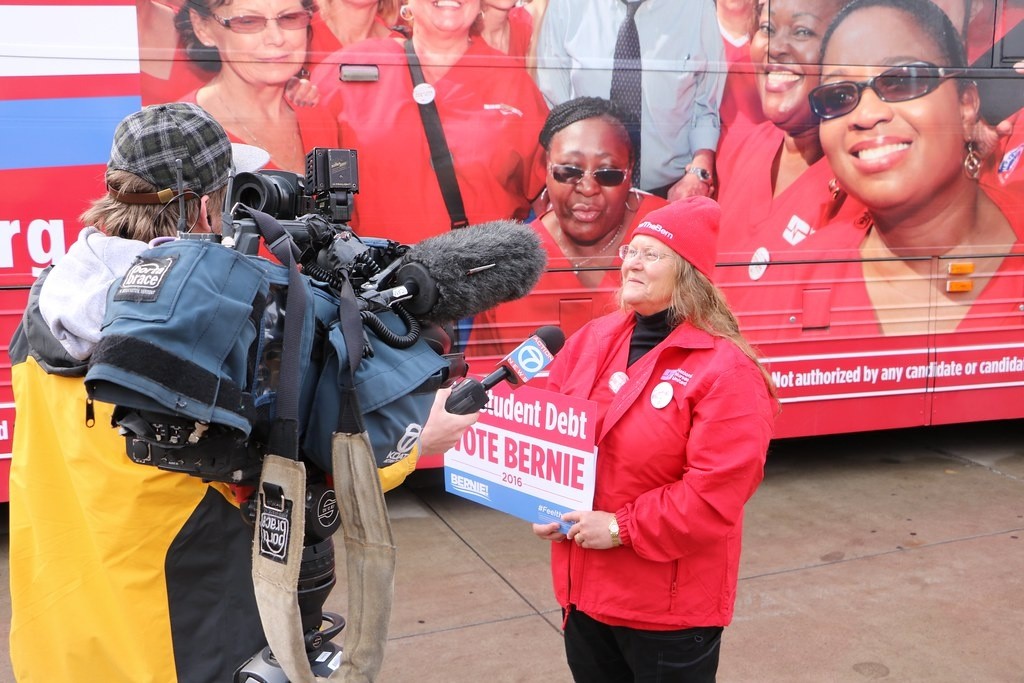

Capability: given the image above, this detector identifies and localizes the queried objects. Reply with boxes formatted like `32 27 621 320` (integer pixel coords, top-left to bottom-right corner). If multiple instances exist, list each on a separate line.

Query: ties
610 0 645 191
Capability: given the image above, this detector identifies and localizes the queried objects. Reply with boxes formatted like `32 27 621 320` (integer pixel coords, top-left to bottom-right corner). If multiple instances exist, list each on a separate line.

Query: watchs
609 514 620 547
688 167 713 188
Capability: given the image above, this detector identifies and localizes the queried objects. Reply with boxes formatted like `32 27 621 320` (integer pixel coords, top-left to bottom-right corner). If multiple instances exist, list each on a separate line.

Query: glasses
547 158 629 186
209 12 314 34
619 244 678 265
808 61 964 120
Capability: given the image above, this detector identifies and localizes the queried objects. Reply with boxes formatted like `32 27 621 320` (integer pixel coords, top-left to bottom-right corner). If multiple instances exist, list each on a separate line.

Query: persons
533 196 782 683
9 101 480 683
137 0 1024 360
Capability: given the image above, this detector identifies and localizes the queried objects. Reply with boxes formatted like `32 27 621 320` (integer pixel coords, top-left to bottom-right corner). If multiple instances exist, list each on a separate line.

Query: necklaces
213 76 297 170
558 225 621 273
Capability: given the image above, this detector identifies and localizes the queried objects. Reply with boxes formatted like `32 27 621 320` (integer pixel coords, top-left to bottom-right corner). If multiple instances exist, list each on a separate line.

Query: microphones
446 327 565 416
393 218 548 327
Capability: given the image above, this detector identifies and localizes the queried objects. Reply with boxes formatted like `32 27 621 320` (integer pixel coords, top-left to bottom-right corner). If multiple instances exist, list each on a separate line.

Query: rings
576 533 582 543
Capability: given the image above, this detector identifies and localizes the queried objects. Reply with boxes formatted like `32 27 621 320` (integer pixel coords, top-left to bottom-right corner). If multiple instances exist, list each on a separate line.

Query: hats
633 195 722 284
104 102 270 205
